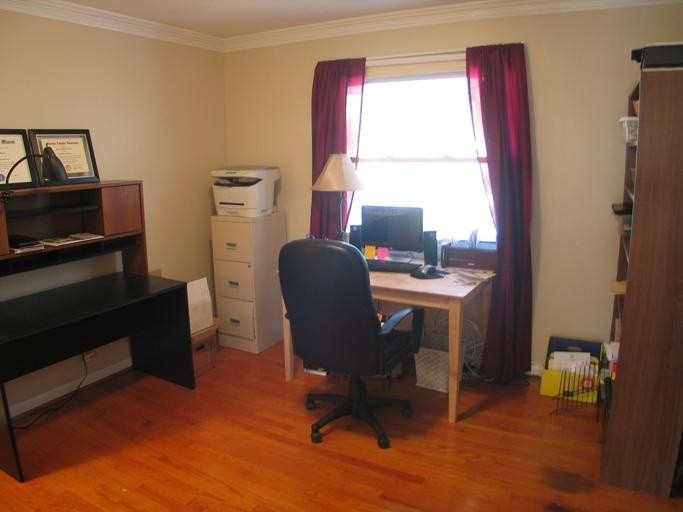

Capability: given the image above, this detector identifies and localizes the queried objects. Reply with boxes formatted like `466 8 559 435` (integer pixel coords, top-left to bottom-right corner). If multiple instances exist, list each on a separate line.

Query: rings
437 270 449 274
307 232 328 240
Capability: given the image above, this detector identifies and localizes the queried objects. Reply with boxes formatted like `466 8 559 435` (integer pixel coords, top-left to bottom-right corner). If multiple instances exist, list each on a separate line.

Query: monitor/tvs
9 231 104 254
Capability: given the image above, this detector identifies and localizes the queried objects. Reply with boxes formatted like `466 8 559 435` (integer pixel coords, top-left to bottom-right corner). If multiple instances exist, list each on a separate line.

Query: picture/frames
0 270 195 481
280 256 494 423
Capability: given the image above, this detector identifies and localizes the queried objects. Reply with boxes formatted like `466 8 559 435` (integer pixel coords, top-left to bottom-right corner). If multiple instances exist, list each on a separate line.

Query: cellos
211 166 280 218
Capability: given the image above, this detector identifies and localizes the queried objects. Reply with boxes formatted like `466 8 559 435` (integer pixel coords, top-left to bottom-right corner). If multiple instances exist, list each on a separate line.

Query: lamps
278 231 425 447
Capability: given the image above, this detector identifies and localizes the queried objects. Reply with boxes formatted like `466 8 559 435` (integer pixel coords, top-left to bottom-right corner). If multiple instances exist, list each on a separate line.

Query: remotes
421 265 436 275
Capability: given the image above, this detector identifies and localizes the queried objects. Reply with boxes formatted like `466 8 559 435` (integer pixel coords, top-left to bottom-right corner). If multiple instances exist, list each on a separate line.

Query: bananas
364 259 421 273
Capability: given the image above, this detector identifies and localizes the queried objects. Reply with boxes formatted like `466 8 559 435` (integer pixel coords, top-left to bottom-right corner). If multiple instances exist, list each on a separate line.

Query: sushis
8 234 38 249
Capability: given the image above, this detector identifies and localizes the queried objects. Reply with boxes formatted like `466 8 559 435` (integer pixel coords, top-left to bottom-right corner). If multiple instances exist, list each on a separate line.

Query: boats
423 231 437 267
349 225 361 252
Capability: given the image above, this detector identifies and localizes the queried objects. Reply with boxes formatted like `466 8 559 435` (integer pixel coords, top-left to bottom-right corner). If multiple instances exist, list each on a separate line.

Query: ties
361 206 423 262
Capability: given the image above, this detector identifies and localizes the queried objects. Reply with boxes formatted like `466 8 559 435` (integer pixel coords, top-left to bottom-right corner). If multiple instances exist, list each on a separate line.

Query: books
190 316 223 376
538 364 599 405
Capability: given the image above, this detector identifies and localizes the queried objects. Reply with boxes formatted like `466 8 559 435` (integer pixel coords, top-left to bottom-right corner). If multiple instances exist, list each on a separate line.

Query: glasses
313 153 357 238
2 148 65 191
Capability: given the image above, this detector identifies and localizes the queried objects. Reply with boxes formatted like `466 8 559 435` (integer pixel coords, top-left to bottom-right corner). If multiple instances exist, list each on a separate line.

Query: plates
209 214 286 356
600 67 683 499
0 179 149 279
